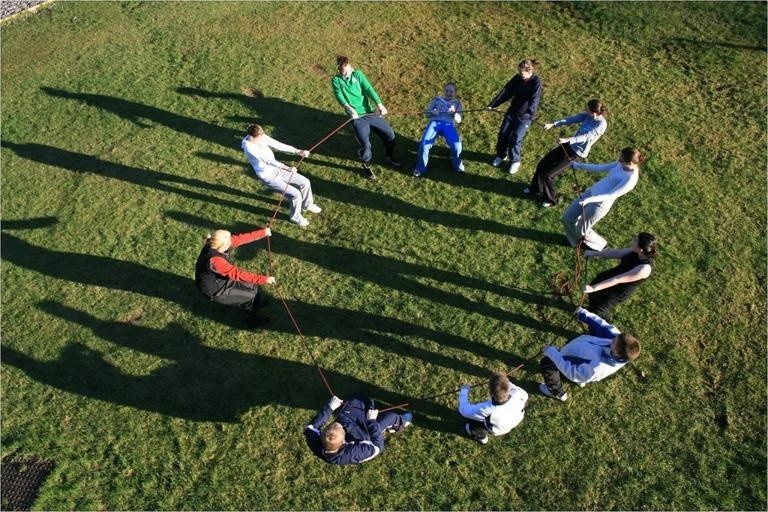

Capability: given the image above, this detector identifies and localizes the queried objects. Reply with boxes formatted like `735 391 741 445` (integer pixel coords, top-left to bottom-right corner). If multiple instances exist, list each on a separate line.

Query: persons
195 227 276 326
334 55 404 180
303 395 413 464
242 124 322 226
412 82 468 177
582 232 657 325
523 99 608 208
538 306 642 402
487 58 541 175
561 147 645 252
458 372 529 444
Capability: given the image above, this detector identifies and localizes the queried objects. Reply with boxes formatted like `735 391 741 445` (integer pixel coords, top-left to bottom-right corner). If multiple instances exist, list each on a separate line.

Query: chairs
384 157 401 167
287 216 309 229
362 164 378 181
538 383 567 401
491 155 508 168
302 205 323 214
464 423 489 444
508 162 523 175
402 414 413 431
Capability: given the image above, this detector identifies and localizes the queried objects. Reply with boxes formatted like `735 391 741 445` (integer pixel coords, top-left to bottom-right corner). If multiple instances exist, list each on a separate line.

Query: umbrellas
247 313 271 328
256 299 270 308
